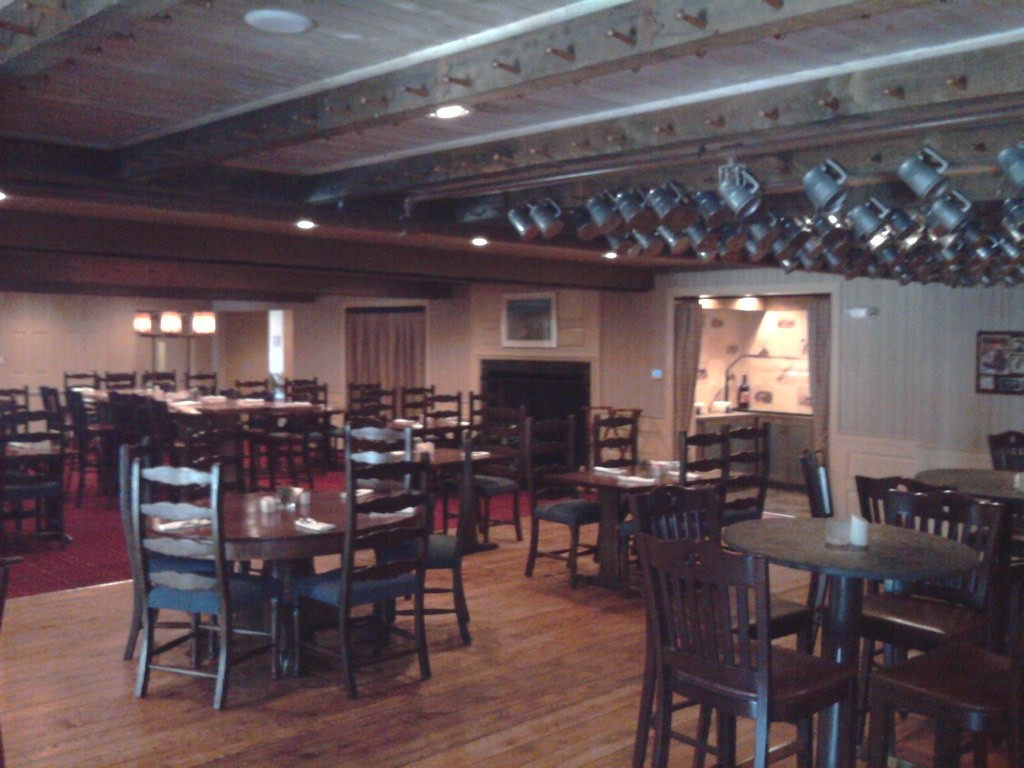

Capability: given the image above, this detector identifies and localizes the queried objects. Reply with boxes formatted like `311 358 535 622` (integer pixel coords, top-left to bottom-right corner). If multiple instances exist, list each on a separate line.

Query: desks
3 441 82 542
545 466 722 591
190 400 345 495
384 443 517 533
916 467 1024 653
723 518 978 768
143 484 425 678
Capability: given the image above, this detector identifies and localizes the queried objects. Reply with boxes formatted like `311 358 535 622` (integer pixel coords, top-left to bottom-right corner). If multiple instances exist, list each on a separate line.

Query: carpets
0 431 599 602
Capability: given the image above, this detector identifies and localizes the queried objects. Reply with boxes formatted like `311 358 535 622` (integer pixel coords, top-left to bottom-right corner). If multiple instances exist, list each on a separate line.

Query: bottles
737 374 750 409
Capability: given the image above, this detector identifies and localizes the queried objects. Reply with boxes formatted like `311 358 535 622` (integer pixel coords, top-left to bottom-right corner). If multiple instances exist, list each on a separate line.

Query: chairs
0 372 533 710
520 399 1024 767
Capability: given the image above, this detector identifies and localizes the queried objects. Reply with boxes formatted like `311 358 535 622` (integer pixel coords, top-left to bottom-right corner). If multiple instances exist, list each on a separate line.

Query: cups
825 516 850 549
850 515 869 546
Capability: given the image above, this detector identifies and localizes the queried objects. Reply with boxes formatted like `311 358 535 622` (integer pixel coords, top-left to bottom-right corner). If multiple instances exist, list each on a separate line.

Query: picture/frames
498 291 557 349
974 331 1024 396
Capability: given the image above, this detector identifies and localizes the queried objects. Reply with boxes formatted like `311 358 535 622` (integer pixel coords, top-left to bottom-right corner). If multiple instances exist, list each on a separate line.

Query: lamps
801 158 852 217
528 195 566 239
896 145 949 202
575 178 1024 288
506 207 537 240
849 197 889 239
718 165 762 223
132 309 215 338
932 189 972 234
996 146 1024 185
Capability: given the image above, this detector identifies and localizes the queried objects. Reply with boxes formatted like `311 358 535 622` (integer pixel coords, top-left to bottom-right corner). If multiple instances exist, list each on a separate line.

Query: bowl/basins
667 470 700 483
